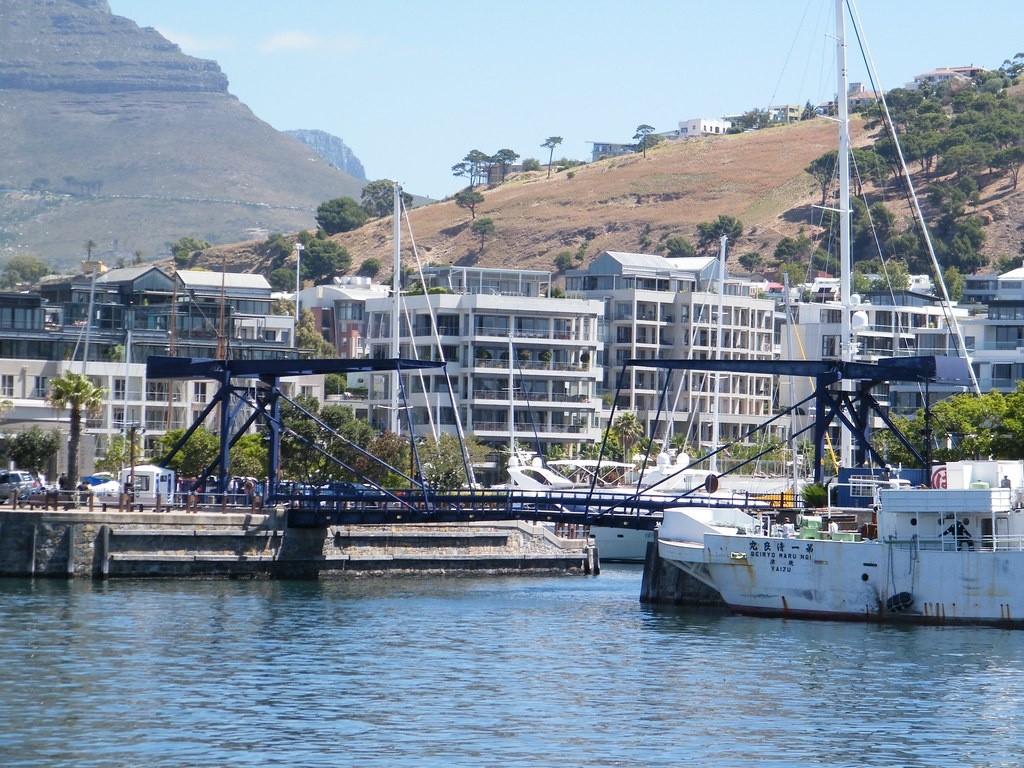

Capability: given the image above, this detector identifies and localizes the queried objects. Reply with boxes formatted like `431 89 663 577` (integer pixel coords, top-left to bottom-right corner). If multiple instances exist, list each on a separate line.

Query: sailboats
534 235 803 561
637 2 1023 623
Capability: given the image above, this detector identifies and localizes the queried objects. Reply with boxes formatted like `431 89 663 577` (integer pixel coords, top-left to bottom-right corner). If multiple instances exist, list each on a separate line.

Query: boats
0 461 387 509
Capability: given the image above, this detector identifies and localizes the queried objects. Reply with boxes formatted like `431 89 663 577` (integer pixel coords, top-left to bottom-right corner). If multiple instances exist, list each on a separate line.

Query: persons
777 518 791 538
58 471 68 489
938 520 976 551
877 467 891 491
827 517 838 541
762 518 771 536
77 480 93 505
